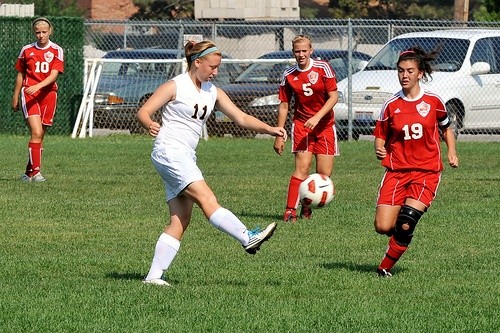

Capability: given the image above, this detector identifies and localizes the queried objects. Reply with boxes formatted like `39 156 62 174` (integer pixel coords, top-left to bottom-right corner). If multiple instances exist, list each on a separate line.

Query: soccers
298 173 337 209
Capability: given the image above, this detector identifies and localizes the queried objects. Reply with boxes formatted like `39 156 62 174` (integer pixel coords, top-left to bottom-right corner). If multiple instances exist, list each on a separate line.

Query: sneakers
21 172 46 182
284 211 296 222
142 279 170 287
242 222 277 255
300 207 311 220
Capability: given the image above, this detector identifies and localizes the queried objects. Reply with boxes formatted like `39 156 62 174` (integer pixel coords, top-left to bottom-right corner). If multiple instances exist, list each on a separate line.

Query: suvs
332 29 499 141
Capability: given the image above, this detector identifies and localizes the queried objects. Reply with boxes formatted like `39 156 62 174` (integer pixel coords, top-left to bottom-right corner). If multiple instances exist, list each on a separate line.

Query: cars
207 50 385 139
82 49 245 135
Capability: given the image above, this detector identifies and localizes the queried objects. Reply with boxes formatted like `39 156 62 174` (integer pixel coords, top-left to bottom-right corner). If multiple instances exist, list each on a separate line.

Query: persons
372 46 458 277
274 35 339 220
12 17 64 182
137 41 288 288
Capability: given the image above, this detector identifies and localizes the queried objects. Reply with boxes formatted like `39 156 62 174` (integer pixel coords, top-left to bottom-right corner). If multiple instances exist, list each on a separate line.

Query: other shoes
386 230 392 237
376 267 392 278
